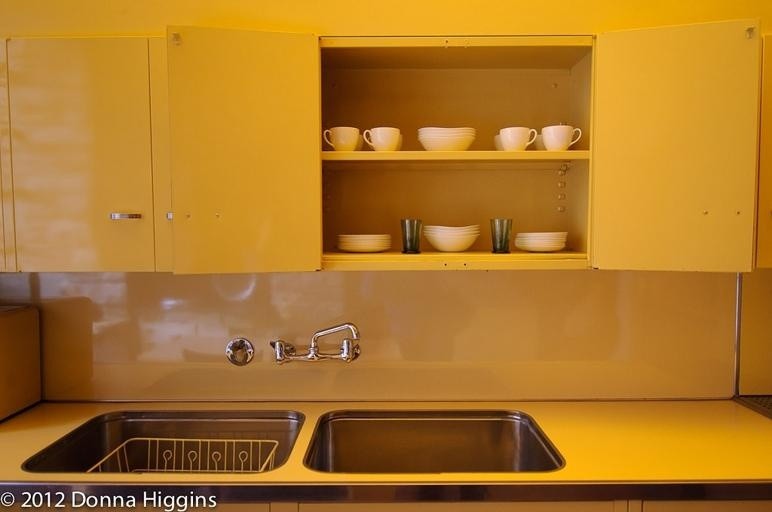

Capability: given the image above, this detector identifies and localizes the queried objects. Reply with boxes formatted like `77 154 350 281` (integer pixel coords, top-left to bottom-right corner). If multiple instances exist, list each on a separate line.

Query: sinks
299 407 568 475
20 409 307 477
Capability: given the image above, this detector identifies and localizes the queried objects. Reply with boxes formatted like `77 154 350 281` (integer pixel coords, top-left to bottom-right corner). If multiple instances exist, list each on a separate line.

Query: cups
490 216 513 255
534 134 546 151
541 124 583 153
322 125 360 151
366 134 403 151
354 135 363 152
400 217 423 254
362 127 400 152
494 134 504 150
499 126 537 151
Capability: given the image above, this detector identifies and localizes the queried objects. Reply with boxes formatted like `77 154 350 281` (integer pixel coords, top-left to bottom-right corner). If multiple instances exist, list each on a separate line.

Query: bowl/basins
423 221 481 253
416 125 476 151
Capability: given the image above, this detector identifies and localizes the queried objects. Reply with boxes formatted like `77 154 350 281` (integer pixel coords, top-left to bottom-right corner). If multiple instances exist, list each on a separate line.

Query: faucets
269 321 361 365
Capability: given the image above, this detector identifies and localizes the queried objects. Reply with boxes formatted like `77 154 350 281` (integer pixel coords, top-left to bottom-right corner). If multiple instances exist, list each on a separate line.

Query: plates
333 233 392 254
514 228 570 253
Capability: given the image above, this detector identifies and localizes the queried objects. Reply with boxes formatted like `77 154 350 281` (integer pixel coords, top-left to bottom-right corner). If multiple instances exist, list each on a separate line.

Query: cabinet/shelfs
153 19 763 273
5 39 172 273
756 30 770 270
